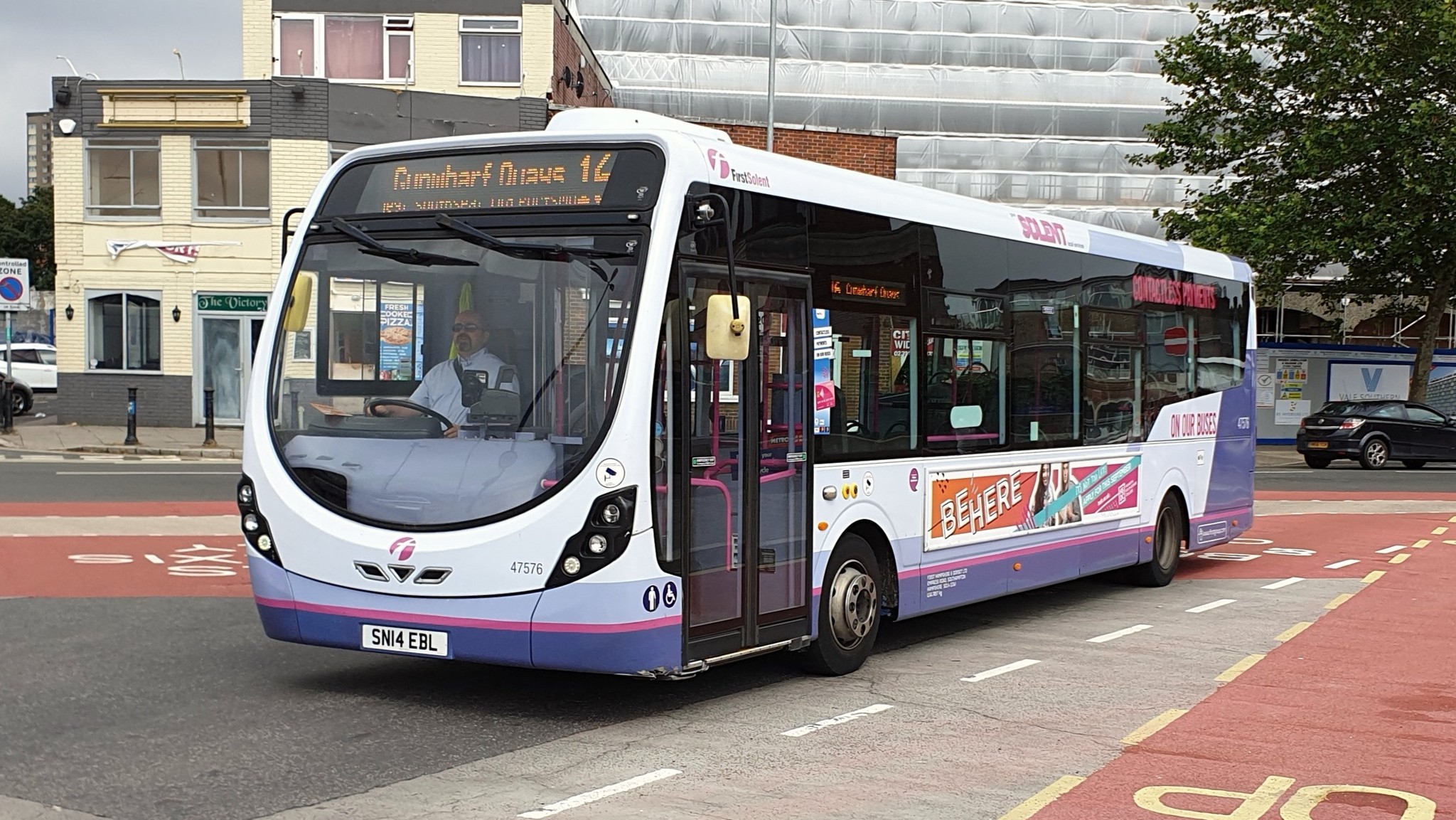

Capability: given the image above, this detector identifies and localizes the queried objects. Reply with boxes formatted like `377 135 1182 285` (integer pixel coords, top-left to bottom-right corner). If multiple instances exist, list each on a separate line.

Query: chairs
847 360 1211 440
484 328 531 427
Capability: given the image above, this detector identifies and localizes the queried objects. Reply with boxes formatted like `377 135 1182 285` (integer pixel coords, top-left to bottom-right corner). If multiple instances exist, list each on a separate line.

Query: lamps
558 67 582 88
272 80 306 93
580 54 588 69
66 304 74 320
173 305 181 322
56 77 73 107
572 71 597 98
59 118 77 134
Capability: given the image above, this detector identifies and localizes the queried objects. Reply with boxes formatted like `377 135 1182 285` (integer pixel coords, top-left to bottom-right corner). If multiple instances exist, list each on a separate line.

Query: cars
0 371 35 416
0 343 57 388
1296 400 1456 471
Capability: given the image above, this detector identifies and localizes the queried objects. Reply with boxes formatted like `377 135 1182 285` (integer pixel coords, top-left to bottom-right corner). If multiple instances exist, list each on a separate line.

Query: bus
233 108 1258 682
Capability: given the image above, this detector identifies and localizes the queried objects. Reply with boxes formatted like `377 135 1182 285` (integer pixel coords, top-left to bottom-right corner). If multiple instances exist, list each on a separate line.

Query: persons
366 307 521 437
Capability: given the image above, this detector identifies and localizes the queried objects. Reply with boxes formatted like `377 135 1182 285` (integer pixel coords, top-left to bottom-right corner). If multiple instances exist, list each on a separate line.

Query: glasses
452 323 478 333
